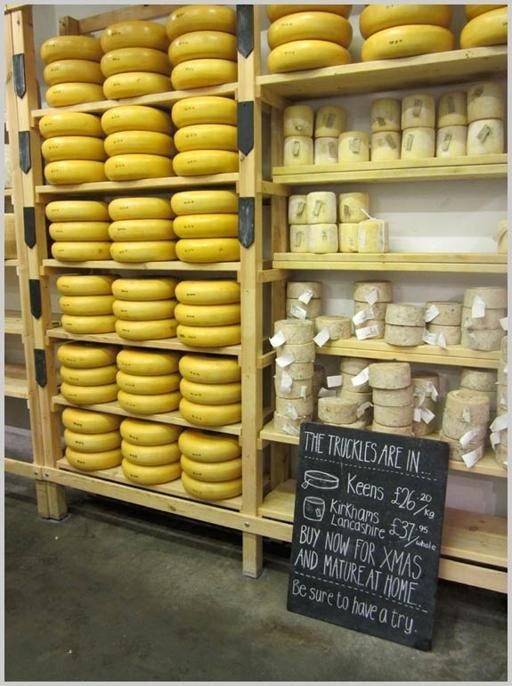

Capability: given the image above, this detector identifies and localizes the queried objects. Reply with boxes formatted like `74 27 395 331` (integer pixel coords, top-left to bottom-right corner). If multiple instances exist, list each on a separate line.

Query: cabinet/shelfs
1 1 245 578
245 1 509 602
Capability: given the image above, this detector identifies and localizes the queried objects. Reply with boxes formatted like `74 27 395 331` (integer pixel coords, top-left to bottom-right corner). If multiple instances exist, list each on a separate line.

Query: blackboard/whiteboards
286 422 450 652
